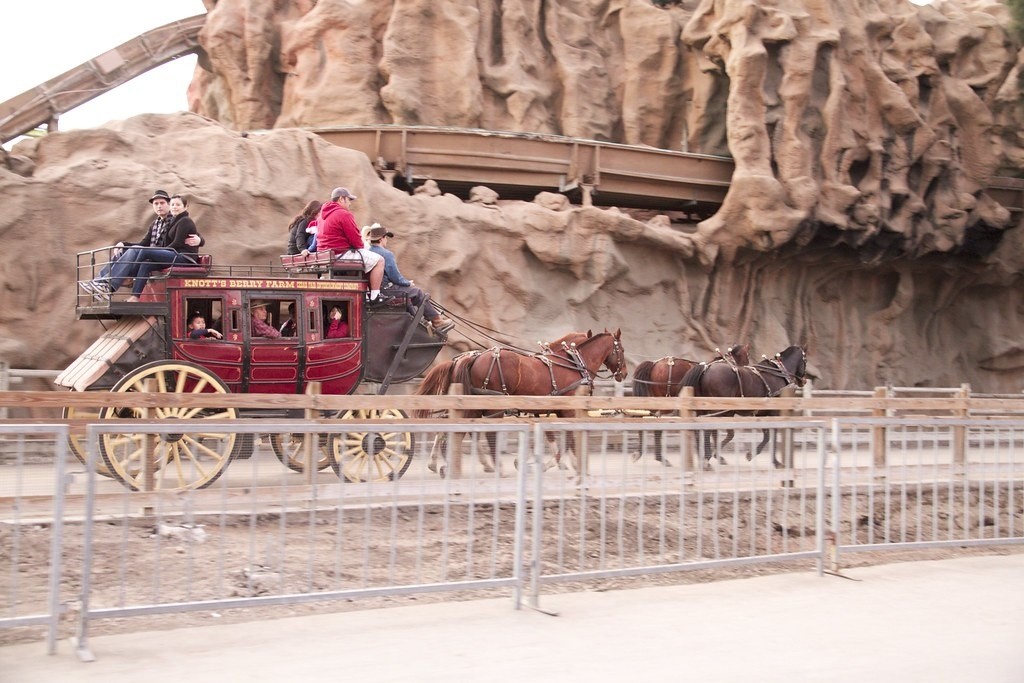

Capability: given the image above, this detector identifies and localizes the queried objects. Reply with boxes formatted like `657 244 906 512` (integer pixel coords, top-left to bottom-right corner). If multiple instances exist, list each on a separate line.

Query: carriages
51 244 808 489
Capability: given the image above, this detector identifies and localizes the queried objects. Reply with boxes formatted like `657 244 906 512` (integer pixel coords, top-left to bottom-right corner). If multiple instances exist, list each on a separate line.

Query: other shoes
127 296 139 302
78 281 105 302
370 293 396 308
89 281 111 301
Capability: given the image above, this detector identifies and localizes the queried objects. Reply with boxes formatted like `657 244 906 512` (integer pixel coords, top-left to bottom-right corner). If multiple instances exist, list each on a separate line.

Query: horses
456 328 627 475
678 344 809 469
632 342 753 472
414 328 593 475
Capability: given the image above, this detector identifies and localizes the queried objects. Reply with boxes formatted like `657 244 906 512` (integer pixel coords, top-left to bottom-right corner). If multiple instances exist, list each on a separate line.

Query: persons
120 194 199 302
251 298 349 339
187 313 224 341
78 188 206 301
287 187 396 303
361 222 452 330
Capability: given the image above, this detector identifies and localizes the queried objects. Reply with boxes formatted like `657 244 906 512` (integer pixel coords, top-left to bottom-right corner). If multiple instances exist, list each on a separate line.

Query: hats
332 188 357 201
369 226 394 240
251 299 271 308
148 190 171 204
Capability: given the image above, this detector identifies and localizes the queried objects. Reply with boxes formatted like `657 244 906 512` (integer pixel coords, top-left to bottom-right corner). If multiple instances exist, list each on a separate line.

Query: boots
432 316 452 331
